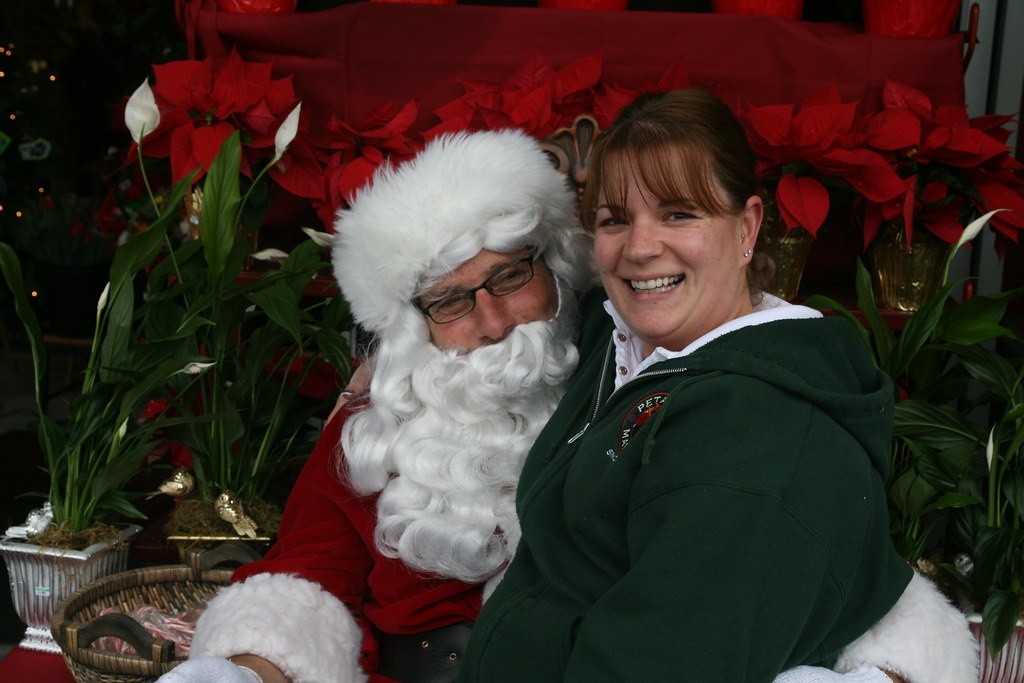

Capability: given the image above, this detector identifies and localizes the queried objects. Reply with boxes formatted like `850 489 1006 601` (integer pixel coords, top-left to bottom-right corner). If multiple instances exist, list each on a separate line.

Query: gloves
153 656 264 683
771 665 894 683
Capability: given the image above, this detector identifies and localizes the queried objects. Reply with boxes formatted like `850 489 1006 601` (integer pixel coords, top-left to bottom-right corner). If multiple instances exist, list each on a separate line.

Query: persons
319 87 915 682
156 129 980 683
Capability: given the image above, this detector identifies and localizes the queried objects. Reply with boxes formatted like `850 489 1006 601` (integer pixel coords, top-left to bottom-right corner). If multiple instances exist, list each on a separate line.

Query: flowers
0 48 1024 621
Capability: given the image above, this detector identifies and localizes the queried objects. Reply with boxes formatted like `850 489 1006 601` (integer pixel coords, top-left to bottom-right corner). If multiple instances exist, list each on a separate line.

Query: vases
0 524 141 654
166 509 275 564
962 613 1024 683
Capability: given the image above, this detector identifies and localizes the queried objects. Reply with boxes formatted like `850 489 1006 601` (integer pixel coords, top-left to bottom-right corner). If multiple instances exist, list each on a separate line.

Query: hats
333 129 589 333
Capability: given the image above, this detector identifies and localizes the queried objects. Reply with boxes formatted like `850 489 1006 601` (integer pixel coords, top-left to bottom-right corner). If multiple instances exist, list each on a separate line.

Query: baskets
50 549 244 683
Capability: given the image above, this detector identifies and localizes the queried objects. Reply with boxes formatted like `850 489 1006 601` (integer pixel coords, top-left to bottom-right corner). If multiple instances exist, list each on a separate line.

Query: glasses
413 251 534 325
